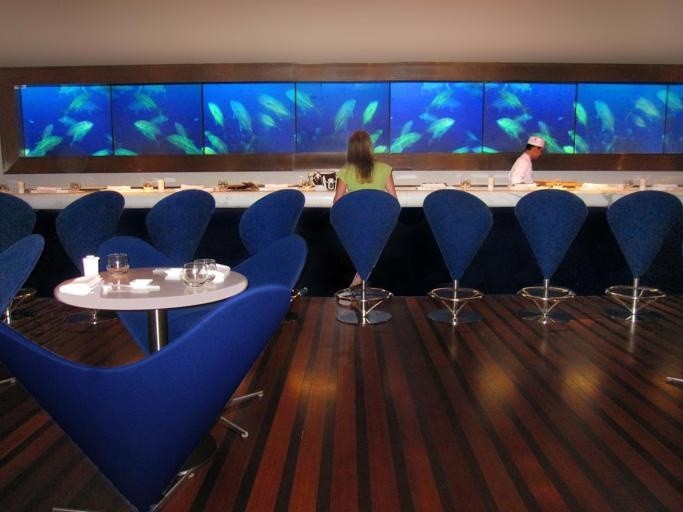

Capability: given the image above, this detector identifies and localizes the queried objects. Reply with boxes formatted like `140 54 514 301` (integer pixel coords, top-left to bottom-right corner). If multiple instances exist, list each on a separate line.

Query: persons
332 130 397 290
507 136 547 186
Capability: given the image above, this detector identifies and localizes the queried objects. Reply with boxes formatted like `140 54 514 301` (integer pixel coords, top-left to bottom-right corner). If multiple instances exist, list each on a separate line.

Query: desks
56 266 248 351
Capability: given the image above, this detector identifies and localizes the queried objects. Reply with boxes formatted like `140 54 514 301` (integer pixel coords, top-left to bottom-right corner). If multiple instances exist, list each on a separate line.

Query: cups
217 176 229 191
16 180 25 195
624 178 635 188
157 176 165 192
182 257 216 286
487 174 494 191
70 181 81 192
460 175 471 189
638 176 646 191
104 253 132 277
142 180 153 191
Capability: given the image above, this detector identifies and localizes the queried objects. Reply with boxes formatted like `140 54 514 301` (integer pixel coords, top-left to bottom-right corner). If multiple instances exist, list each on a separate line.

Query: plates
164 267 183 276
216 262 231 271
129 278 153 286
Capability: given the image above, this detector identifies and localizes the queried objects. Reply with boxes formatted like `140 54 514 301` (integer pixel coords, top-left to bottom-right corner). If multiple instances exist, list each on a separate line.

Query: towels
59 275 102 295
203 261 230 283
152 266 182 281
125 277 160 294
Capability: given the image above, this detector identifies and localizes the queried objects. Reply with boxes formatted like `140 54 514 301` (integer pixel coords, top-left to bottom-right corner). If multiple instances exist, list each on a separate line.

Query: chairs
96 235 306 437
145 185 215 265
329 189 400 325
515 187 589 325
0 192 39 303
1 232 45 322
1 284 291 510
603 188 681 322
422 190 494 325
236 188 308 324
53 191 124 324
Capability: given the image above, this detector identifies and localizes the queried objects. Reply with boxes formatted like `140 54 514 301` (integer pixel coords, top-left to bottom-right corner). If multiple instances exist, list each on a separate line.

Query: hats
527 136 544 147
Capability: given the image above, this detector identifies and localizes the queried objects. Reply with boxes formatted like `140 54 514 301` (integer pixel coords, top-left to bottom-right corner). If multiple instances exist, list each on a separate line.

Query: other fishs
15 82 683 158
